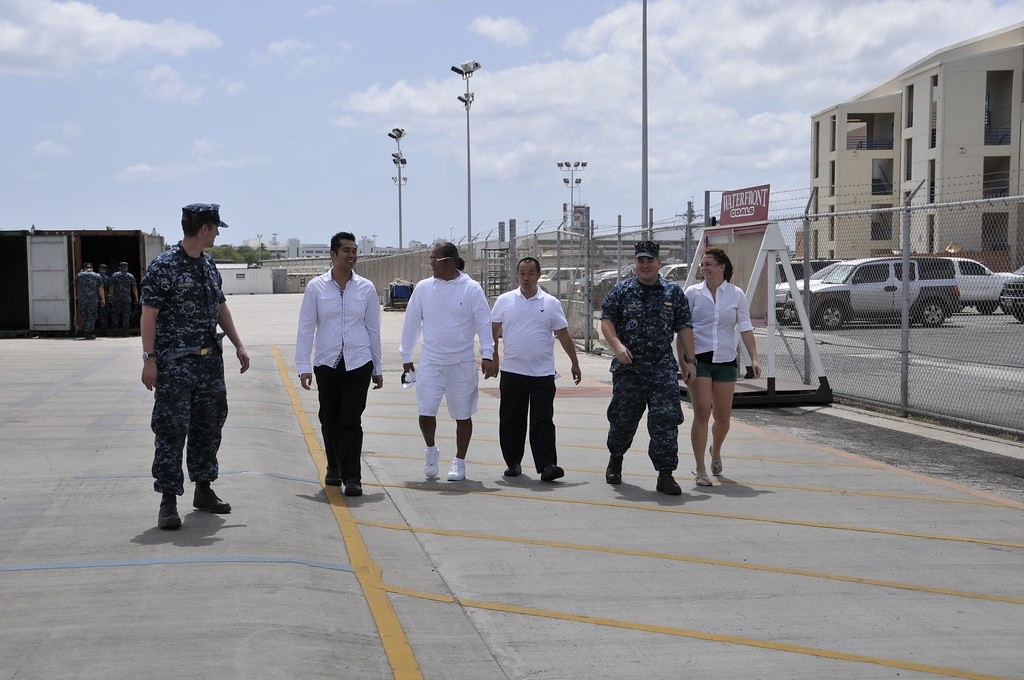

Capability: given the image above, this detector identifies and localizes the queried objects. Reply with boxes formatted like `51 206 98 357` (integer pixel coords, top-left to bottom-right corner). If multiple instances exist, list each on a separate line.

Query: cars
1000 281 1024 324
539 263 637 308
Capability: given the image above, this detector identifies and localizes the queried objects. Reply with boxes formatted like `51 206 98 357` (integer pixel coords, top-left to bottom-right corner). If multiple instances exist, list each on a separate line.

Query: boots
158 494 182 529
193 481 232 512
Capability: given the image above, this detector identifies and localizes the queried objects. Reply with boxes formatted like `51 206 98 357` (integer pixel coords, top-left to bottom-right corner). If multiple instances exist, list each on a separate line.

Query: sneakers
424 447 440 478
447 460 465 481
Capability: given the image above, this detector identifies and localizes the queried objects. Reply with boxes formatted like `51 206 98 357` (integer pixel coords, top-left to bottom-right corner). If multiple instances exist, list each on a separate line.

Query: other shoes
86 328 129 339
656 475 682 495
606 454 624 484
325 467 342 485
345 482 363 496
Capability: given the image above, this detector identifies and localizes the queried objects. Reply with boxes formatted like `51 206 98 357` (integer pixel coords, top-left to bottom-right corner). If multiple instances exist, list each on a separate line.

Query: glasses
429 257 451 262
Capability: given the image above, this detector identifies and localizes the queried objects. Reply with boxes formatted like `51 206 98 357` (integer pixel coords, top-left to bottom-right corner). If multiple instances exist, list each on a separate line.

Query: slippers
709 445 723 475
695 474 713 486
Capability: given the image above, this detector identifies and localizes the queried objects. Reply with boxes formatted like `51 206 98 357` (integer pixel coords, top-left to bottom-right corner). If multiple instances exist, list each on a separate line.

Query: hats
182 202 228 228
634 241 660 258
99 264 108 268
120 262 129 267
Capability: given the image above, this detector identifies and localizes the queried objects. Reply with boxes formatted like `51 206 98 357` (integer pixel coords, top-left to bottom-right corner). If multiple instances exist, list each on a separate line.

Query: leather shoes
504 465 522 476
541 464 565 481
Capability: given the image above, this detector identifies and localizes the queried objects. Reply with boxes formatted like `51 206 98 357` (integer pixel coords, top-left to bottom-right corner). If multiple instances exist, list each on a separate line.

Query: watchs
686 358 697 365
142 352 156 361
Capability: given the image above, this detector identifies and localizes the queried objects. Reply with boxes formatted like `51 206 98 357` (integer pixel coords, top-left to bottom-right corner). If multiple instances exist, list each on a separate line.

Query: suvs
776 259 843 321
785 256 963 327
659 264 702 290
947 257 1023 315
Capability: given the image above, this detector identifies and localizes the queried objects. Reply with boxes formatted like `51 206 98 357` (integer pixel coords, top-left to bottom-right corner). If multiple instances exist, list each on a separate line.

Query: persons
74 262 106 340
601 239 697 495
96 261 139 335
141 203 250 529
398 242 496 482
490 257 581 481
675 248 761 488
296 232 383 496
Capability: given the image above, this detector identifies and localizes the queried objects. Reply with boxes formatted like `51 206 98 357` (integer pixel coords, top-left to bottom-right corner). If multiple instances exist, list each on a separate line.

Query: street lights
388 127 407 253
257 234 263 262
451 61 481 279
557 161 587 264
